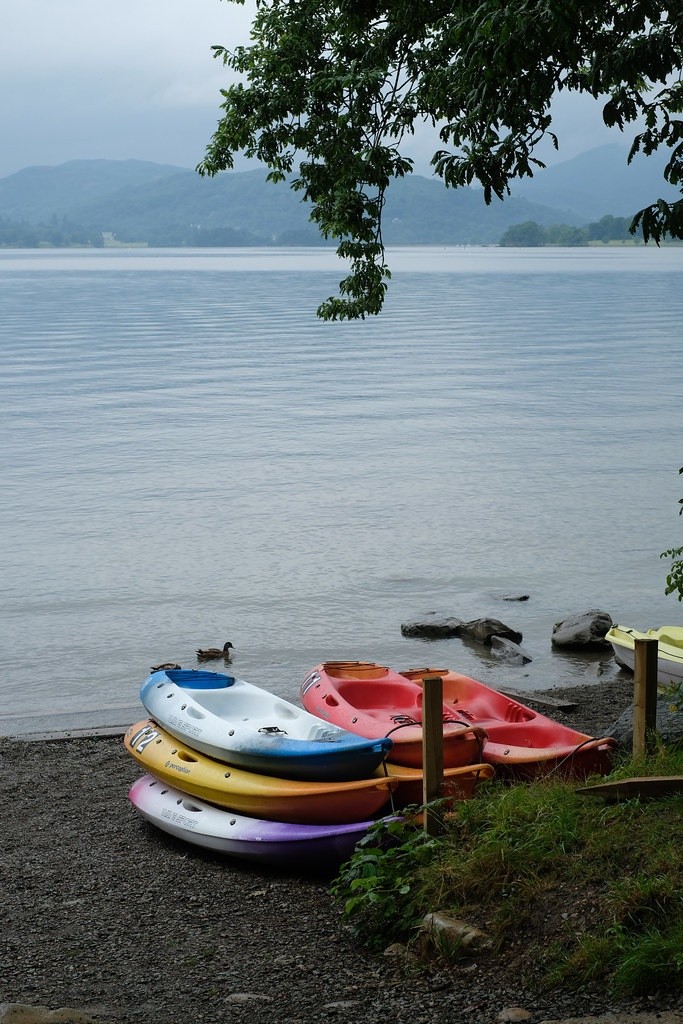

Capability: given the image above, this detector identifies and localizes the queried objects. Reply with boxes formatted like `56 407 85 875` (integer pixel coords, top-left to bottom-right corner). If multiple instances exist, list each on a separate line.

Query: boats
123 719 400 825
128 774 405 863
606 621 683 691
139 668 395 781
398 666 621 784
372 755 496 823
299 661 489 770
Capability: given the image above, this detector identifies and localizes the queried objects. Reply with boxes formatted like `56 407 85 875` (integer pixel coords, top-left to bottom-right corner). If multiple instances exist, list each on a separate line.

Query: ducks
150 662 184 675
195 642 236 663
